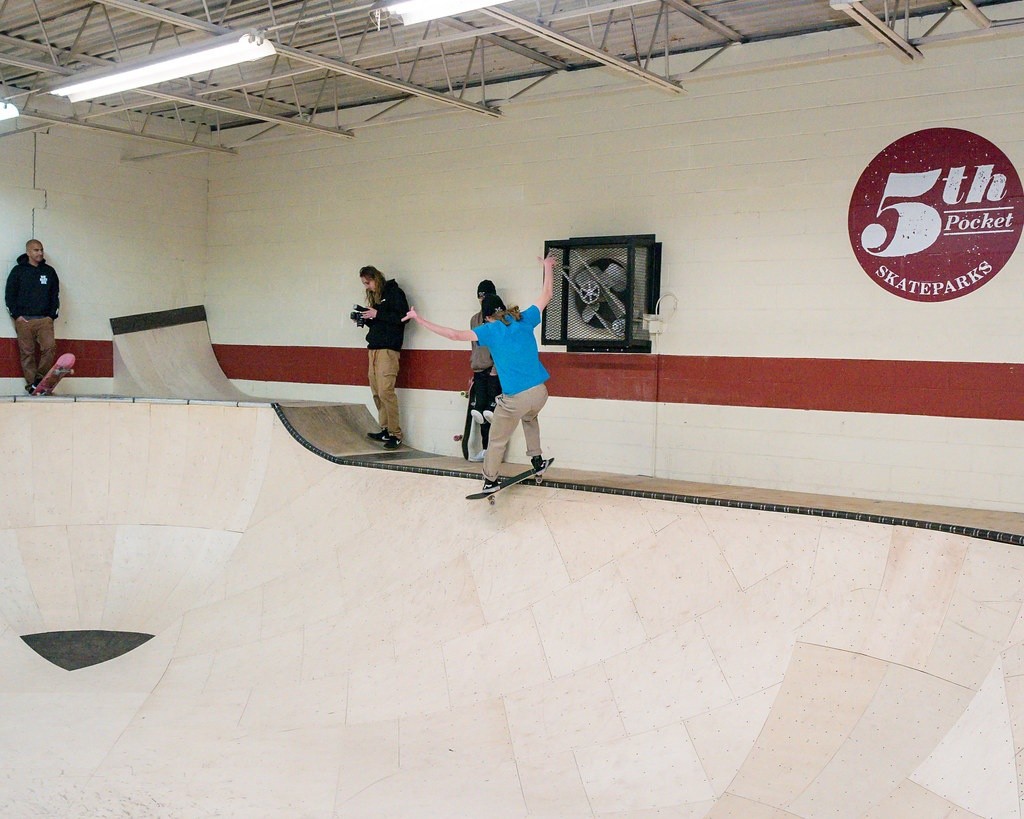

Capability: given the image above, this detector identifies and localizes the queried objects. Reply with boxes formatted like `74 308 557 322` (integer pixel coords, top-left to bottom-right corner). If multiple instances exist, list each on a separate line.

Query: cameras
351 311 369 328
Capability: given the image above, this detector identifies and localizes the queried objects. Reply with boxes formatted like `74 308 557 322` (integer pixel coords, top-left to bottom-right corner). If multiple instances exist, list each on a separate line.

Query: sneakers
531 458 549 475
468 450 487 462
367 429 390 442
383 436 402 450
482 478 500 493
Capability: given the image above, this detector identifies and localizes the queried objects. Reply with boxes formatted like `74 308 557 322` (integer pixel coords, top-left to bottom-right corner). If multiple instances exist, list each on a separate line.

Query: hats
477 279 496 298
482 294 506 319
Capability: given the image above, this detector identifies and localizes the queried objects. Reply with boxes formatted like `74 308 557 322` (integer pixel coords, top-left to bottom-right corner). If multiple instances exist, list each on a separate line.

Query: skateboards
452 382 476 460
465 458 556 505
29 353 76 396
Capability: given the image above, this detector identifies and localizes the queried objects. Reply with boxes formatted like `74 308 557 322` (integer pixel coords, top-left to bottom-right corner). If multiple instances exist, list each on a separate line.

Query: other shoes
29 375 44 394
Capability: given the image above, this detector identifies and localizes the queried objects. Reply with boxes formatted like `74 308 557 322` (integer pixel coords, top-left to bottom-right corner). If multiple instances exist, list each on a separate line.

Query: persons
470 281 502 463
359 266 411 451
5 240 60 394
401 251 557 493
